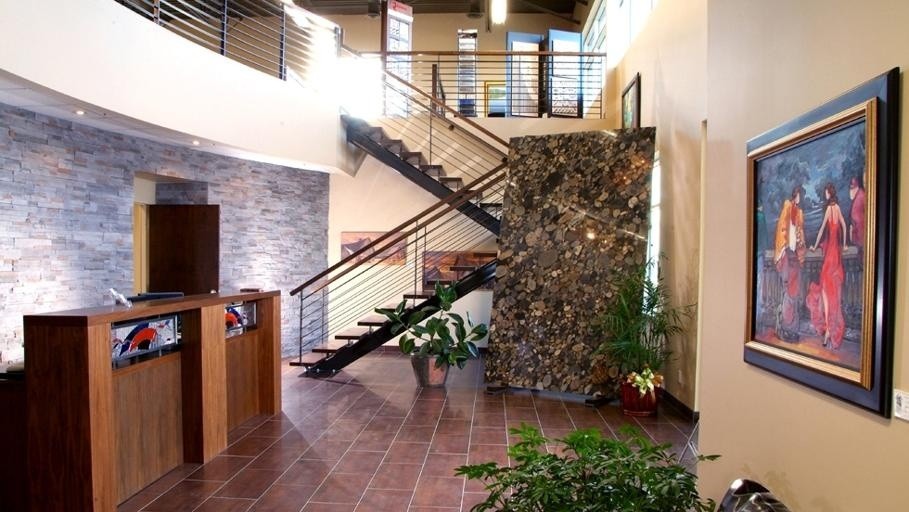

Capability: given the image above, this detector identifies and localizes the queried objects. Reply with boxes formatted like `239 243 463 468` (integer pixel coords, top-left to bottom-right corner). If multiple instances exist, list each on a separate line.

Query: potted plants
593 251 695 418
374 283 488 388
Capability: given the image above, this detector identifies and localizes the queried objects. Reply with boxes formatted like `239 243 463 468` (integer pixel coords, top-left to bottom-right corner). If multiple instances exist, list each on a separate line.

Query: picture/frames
620 72 641 129
483 79 506 118
422 250 498 293
742 65 902 422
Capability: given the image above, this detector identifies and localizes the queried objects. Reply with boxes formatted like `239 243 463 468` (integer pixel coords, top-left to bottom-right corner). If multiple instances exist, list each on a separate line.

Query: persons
773 186 806 343
806 183 849 350
756 198 768 330
850 177 866 269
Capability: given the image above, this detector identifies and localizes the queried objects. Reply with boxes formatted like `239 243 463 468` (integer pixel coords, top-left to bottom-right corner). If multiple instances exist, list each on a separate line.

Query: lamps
367 1 380 18
466 0 511 33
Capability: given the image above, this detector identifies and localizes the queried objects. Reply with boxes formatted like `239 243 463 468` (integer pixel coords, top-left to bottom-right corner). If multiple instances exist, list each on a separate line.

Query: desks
22 288 284 511
